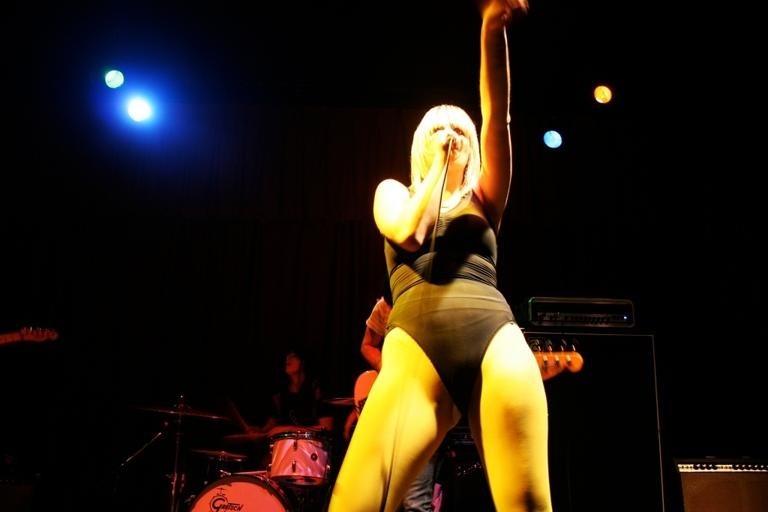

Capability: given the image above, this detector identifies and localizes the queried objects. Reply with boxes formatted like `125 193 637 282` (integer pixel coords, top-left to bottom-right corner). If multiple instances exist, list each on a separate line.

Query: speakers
432 441 497 511
663 455 768 512
523 332 666 512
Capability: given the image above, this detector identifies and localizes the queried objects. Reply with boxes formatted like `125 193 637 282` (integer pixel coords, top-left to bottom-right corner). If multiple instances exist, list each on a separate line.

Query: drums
192 471 289 512
271 429 330 486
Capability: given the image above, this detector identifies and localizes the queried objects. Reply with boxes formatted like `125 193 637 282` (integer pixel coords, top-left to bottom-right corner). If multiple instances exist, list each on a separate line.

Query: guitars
353 337 584 429
0 327 59 344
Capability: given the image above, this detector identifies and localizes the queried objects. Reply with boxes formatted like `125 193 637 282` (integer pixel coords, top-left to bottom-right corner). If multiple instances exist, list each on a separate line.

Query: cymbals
197 447 246 460
149 406 226 421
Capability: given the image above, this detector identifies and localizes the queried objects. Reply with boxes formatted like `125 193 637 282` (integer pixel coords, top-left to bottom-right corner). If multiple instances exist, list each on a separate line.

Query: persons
261 348 323 426
327 1 552 512
360 294 450 512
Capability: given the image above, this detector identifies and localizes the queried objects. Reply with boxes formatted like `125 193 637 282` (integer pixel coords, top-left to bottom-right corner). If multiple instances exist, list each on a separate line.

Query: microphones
447 136 455 149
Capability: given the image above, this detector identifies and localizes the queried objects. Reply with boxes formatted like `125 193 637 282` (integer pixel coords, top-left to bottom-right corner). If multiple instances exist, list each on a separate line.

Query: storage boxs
675 458 768 512
